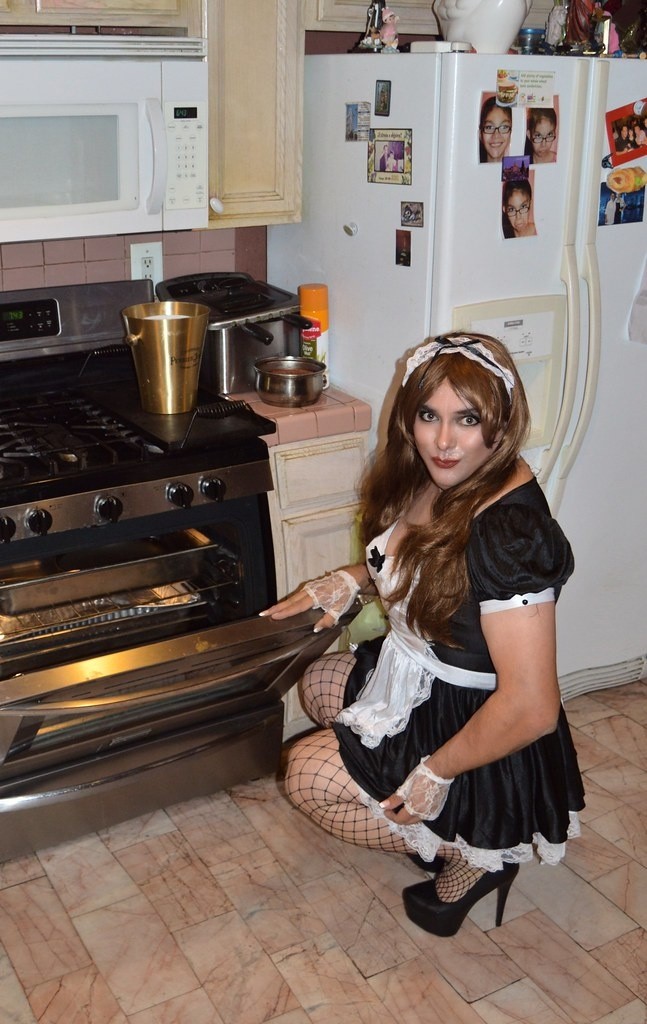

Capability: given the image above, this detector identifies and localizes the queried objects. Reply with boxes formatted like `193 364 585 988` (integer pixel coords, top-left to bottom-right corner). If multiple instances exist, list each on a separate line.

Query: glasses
505 204 530 217
530 133 556 143
482 125 511 134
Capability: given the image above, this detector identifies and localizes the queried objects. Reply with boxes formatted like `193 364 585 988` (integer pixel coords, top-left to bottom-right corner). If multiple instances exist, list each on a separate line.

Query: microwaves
0 33 208 245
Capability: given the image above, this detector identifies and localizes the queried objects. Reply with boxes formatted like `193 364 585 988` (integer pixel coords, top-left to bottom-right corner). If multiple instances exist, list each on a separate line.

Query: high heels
403 853 520 937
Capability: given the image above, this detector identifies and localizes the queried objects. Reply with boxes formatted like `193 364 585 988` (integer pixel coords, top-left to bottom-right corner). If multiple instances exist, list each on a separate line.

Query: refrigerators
266 48 647 698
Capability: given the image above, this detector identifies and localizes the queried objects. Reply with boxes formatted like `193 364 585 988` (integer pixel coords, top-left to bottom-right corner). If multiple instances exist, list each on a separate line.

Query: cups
517 27 546 55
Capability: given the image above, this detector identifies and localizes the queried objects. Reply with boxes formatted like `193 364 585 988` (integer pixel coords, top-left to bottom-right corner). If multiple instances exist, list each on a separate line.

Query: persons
501 180 537 236
387 150 396 171
379 145 389 172
614 118 646 154
481 96 510 162
257 330 586 937
605 192 616 225
614 193 627 225
526 106 557 164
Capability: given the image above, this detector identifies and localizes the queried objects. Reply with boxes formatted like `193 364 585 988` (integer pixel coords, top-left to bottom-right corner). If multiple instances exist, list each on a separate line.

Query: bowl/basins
251 356 328 408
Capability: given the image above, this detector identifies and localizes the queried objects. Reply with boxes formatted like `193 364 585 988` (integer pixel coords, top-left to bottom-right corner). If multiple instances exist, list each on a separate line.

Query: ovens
0 541 361 863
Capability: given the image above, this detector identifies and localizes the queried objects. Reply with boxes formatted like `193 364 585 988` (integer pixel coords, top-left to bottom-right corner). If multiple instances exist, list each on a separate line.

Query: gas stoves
0 276 277 545
